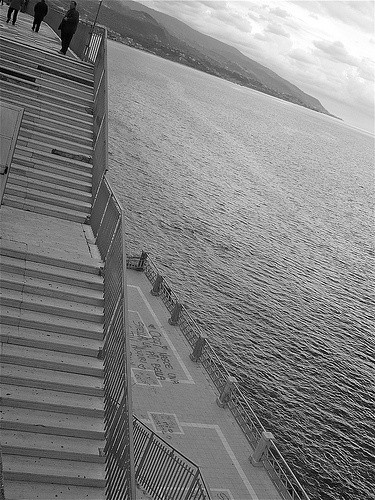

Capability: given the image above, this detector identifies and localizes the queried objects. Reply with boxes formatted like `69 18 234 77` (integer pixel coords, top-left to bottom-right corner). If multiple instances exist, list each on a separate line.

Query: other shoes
35 31 38 33
31 27 34 31
6 20 9 23
57 49 65 54
20 9 24 13
12 22 15 25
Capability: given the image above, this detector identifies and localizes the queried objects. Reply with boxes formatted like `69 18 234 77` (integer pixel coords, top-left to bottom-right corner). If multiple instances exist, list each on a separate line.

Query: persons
56 0 79 55
30 0 48 32
5 0 23 26
